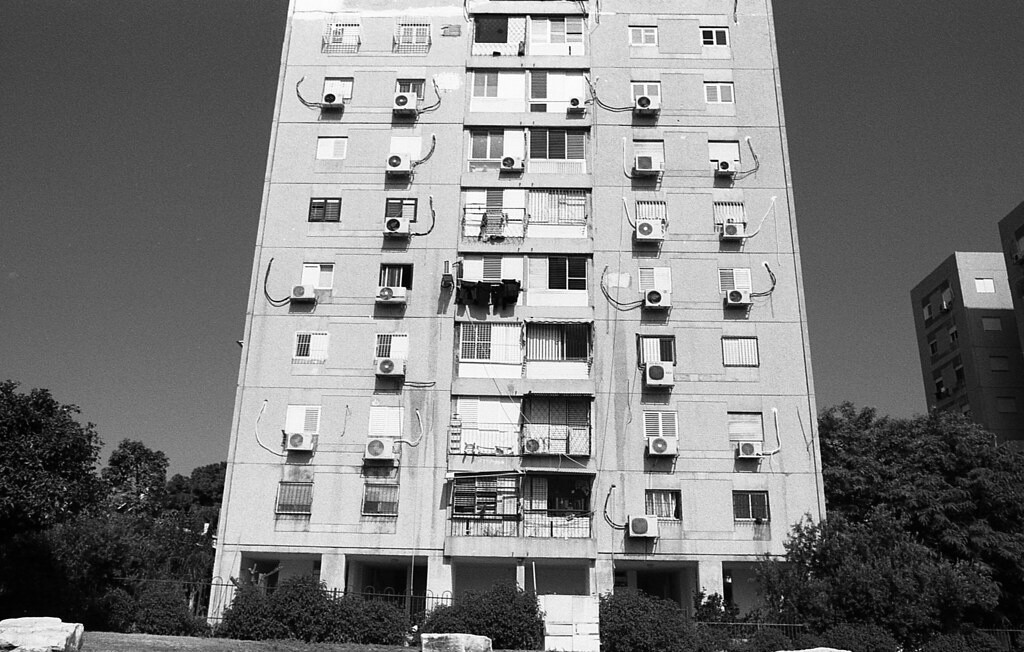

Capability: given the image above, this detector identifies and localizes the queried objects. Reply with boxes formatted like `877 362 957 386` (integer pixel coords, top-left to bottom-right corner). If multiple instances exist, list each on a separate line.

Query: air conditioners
636 95 659 114
501 155 522 172
646 361 673 388
723 223 744 239
635 154 660 175
286 432 313 450
374 286 407 305
726 290 750 306
291 284 315 302
627 515 659 538
718 159 734 175
365 437 393 460
376 357 404 375
321 92 418 239
635 219 662 242
644 289 670 309
524 438 548 453
567 97 584 113
648 436 677 456
738 442 762 458
